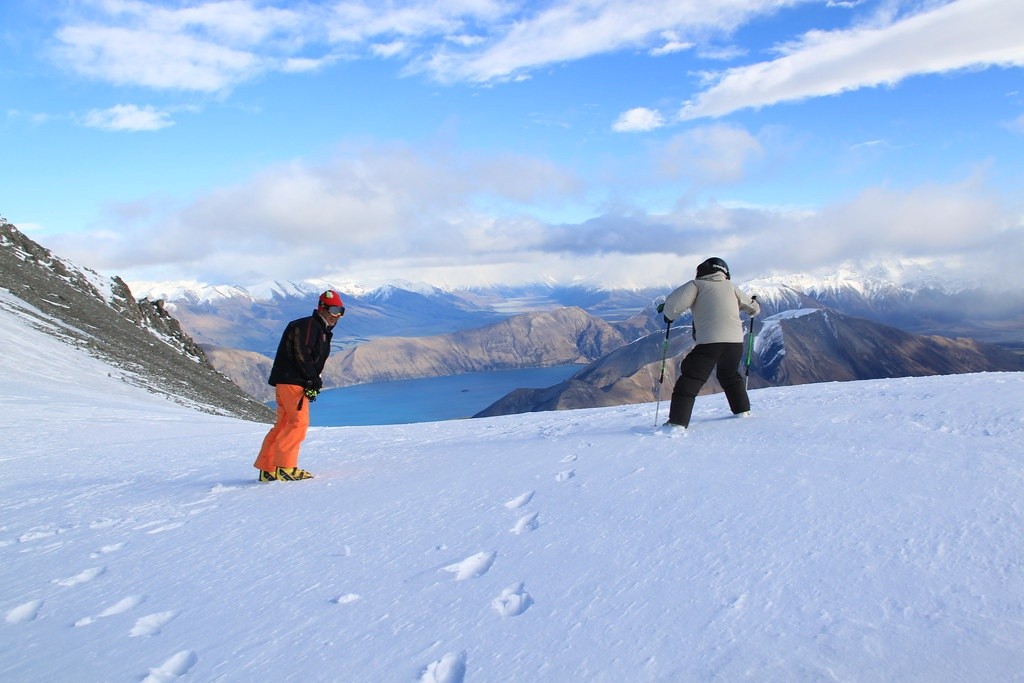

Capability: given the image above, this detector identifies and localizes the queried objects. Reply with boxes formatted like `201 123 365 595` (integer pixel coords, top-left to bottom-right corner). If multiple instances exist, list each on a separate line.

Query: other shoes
275 465 312 481
259 469 276 482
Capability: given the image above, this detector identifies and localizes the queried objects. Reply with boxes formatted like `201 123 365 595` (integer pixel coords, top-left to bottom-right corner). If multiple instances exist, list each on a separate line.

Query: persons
664 257 761 431
253 290 346 483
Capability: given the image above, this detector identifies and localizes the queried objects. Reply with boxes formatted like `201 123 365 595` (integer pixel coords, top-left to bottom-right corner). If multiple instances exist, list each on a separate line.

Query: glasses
328 306 344 317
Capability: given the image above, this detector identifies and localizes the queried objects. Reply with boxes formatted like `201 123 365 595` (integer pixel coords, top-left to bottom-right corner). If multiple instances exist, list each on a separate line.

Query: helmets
318 290 342 313
695 257 729 277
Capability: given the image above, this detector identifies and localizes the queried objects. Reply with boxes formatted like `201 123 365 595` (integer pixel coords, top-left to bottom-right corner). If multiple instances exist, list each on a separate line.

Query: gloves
663 315 674 323
306 379 323 399
